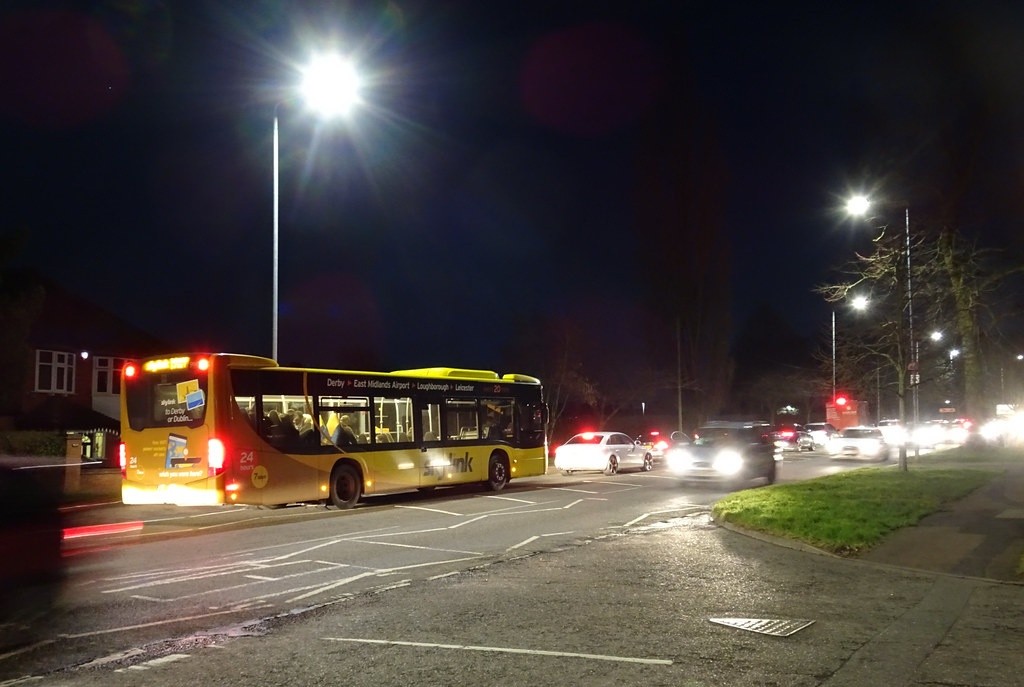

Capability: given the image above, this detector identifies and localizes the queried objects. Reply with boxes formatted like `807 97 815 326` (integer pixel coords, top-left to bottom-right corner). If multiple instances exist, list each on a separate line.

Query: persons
424 432 437 441
241 404 311 434
332 415 357 445
488 417 509 439
376 435 388 443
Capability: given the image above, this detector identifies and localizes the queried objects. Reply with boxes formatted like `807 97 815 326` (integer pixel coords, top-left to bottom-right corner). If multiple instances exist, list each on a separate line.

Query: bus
119 351 554 513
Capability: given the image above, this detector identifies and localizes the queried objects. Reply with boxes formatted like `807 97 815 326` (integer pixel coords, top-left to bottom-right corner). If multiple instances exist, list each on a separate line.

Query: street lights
848 196 921 427
831 296 868 404
950 349 960 365
916 331 942 422
1001 354 1024 398
270 64 362 362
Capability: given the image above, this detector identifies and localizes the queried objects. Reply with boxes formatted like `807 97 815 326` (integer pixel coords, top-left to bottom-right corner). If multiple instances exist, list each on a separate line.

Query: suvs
668 421 778 488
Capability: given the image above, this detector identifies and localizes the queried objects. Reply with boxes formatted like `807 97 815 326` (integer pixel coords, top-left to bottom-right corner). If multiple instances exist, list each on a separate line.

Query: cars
828 417 973 461
804 422 842 443
635 432 670 458
770 423 816 453
555 432 653 477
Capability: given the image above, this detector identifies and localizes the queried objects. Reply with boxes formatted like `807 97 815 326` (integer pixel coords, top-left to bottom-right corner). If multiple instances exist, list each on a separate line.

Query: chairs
268 410 441 448
